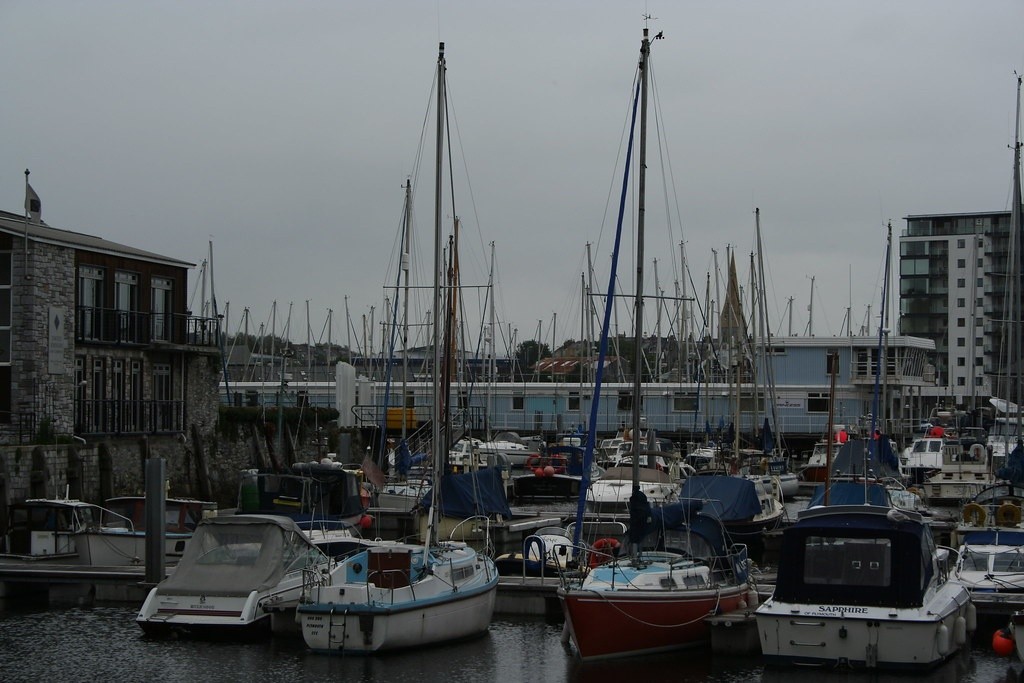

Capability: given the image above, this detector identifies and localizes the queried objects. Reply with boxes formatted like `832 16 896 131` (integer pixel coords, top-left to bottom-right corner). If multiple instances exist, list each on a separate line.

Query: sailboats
3 11 1024 668
